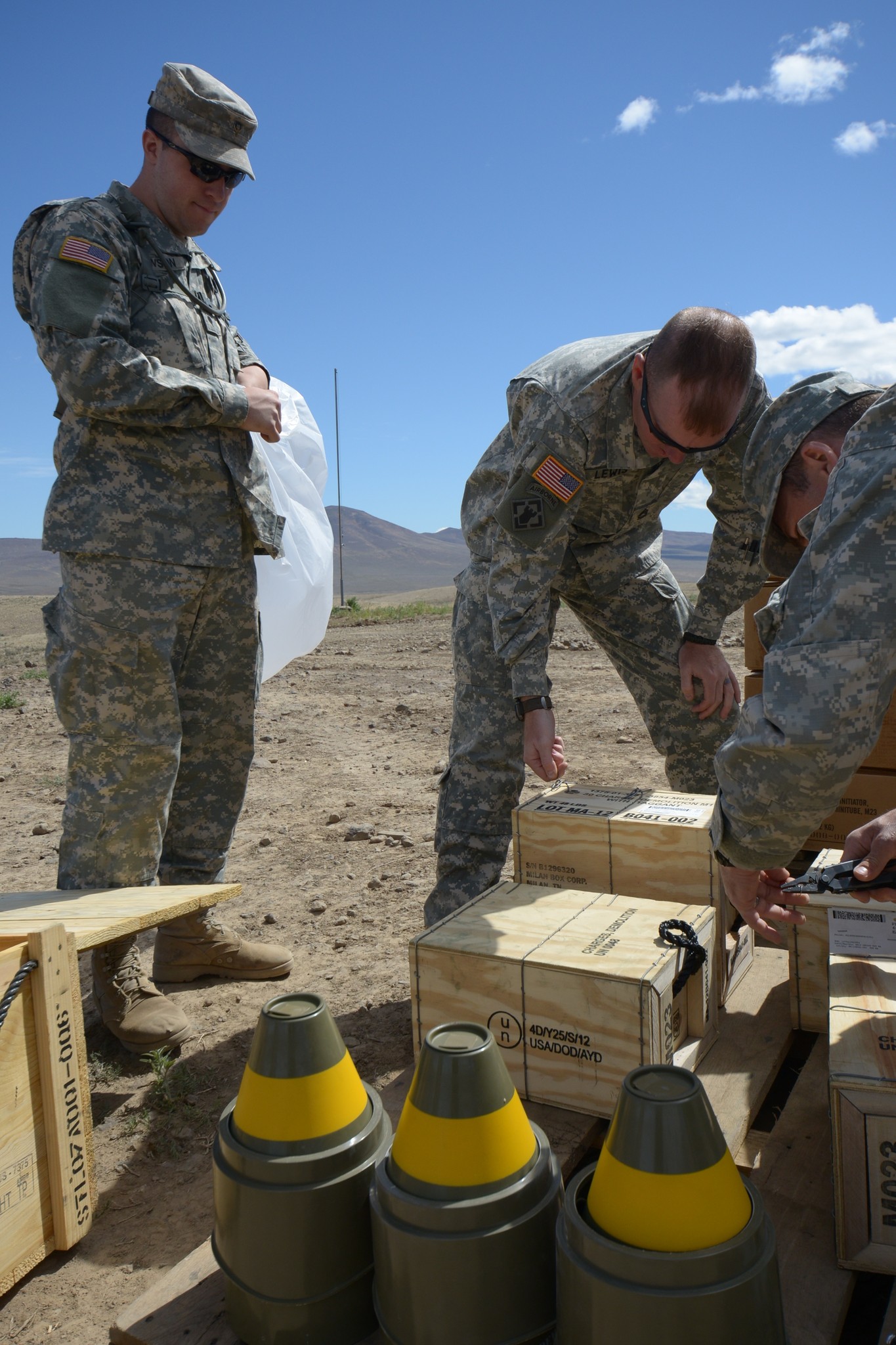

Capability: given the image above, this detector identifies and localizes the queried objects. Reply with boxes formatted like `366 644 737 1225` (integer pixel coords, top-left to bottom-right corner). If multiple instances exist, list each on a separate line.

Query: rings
723 679 732 684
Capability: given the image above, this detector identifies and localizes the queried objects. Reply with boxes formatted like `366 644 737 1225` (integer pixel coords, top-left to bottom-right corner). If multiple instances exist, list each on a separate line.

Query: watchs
514 696 552 722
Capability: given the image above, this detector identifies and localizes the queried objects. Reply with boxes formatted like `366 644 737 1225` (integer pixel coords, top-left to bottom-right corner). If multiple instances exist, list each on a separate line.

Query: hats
742 370 887 579
148 62 259 180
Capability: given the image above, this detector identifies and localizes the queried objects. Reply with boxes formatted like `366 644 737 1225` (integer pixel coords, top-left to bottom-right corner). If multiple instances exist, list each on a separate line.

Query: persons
12 63 294 1056
708 369 896 946
424 304 774 931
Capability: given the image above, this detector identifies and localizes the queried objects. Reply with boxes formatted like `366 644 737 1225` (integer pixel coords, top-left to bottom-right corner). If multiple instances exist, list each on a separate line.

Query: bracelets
685 631 717 646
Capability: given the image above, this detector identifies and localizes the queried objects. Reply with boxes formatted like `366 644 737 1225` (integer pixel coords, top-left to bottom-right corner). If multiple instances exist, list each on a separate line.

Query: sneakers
151 917 295 985
90 943 193 1053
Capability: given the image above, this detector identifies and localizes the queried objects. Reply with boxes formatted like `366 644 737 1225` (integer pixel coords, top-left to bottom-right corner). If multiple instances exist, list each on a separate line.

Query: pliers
779 857 896 895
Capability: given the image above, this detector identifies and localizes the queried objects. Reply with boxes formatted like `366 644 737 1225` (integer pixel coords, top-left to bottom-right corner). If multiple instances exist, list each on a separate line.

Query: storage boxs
745 587 896 852
512 781 754 1005
407 880 720 1122
828 954 896 1275
0 883 243 1296
786 847 896 1034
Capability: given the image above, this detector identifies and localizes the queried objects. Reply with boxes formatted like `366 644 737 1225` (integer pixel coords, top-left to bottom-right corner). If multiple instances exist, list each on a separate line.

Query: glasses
640 343 739 454
145 125 246 189
